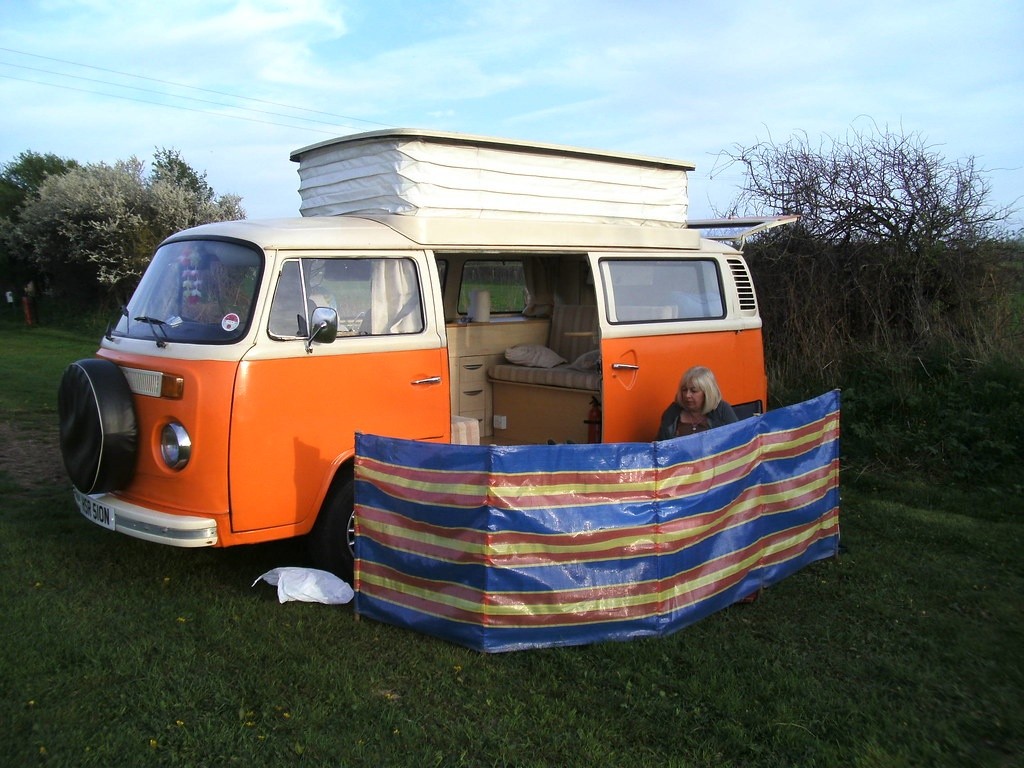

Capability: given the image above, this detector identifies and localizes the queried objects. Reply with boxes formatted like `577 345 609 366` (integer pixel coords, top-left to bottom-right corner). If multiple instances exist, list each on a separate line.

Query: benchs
488 302 679 391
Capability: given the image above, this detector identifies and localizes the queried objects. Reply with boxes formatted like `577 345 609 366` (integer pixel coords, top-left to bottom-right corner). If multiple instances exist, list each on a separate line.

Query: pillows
571 350 601 372
505 342 568 367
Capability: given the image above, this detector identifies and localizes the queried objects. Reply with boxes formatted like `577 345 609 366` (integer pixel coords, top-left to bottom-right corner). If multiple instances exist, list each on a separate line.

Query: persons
653 366 738 441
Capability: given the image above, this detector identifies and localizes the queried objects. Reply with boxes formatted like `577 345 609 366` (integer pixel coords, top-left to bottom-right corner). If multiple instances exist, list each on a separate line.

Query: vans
53 126 802 585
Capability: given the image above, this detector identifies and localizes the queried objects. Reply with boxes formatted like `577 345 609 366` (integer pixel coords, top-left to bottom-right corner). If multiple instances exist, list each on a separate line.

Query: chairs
270 276 317 337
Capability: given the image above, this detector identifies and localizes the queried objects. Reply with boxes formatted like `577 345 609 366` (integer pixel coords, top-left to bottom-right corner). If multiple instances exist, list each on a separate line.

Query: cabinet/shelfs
445 316 552 437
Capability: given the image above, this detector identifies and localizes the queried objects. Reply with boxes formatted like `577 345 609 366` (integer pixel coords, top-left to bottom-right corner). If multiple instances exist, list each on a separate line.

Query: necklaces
687 412 707 431
